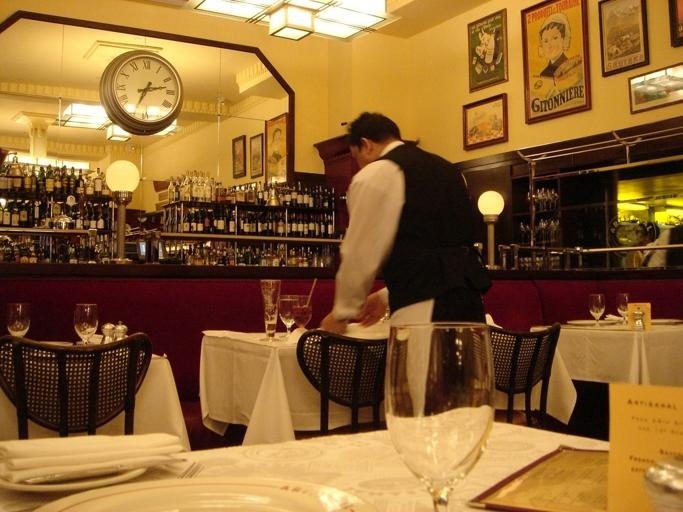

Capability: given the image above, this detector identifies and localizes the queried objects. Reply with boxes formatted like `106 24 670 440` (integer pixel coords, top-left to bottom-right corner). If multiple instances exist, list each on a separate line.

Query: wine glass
614 291 631 330
383 322 497 511
288 294 314 342
519 188 560 243
587 292 603 330
276 294 298 338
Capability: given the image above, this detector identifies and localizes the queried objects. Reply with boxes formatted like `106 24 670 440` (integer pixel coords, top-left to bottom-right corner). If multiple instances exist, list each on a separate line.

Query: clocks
103 50 185 133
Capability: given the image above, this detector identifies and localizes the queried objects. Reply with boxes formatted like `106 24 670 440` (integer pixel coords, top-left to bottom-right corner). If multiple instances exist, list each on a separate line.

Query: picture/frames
231 112 286 187
461 0 683 151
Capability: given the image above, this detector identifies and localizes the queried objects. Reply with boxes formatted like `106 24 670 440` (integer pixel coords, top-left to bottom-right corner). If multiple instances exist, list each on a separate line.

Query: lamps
105 159 141 263
61 103 178 142
476 190 505 269
185 0 388 42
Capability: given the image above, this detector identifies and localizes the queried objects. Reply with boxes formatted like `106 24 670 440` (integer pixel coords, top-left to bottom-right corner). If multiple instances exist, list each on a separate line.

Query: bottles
99 321 128 348
645 203 660 241
630 307 646 332
0 165 111 264
170 166 335 240
178 241 340 270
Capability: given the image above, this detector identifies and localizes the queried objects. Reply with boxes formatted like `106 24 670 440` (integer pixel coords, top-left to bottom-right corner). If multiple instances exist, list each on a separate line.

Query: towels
603 314 623 321
0 433 188 484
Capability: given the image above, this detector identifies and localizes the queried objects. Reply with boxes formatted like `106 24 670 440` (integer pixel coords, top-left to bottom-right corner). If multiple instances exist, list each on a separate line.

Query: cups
257 278 285 343
3 301 31 340
73 303 100 344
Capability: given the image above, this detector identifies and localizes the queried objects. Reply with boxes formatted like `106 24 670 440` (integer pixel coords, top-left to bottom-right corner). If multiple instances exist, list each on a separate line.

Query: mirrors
0 10 296 278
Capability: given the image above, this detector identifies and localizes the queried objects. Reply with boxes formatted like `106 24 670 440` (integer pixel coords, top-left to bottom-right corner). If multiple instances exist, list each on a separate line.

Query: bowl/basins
152 180 169 193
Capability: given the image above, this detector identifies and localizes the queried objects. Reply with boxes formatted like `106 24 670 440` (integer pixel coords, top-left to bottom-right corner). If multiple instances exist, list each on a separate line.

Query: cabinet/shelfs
0 188 114 263
157 200 343 266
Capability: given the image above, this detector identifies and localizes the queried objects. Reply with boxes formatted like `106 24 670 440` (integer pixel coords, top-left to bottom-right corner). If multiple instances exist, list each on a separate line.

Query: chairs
296 329 389 435
489 323 562 431
0 331 152 441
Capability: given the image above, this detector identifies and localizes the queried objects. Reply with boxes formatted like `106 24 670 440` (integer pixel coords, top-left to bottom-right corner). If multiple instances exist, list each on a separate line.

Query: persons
321 112 492 388
637 221 665 267
270 127 281 163
538 13 571 79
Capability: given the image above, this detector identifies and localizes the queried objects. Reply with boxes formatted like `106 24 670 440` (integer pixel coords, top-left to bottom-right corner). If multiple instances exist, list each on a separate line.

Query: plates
0 464 148 495
29 476 373 511
649 318 683 327
567 319 619 327
